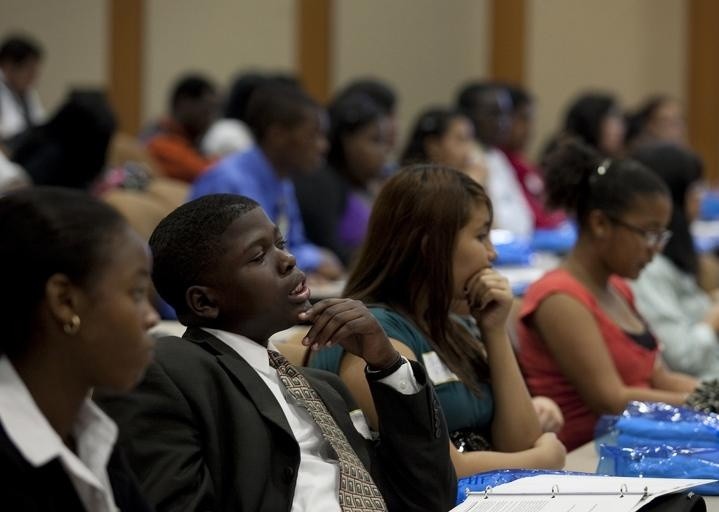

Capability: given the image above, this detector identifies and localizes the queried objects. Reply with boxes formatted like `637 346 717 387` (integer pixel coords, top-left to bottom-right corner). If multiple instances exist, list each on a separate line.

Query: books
448 474 719 512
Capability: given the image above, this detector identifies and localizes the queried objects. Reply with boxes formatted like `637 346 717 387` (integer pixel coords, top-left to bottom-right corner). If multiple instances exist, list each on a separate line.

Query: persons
400 106 477 181
618 142 719 379
107 193 458 512
164 94 343 322
199 69 256 163
457 84 536 234
511 140 699 454
150 73 221 182
549 92 627 159
0 187 160 512
301 164 567 481
299 77 401 268
615 99 718 290
0 33 48 191
492 89 570 231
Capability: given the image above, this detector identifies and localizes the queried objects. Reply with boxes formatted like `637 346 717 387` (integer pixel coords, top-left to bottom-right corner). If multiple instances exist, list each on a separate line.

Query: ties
270 351 387 508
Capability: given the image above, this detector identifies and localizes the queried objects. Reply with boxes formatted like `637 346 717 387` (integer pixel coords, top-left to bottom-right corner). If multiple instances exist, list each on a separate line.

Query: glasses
614 218 673 249
